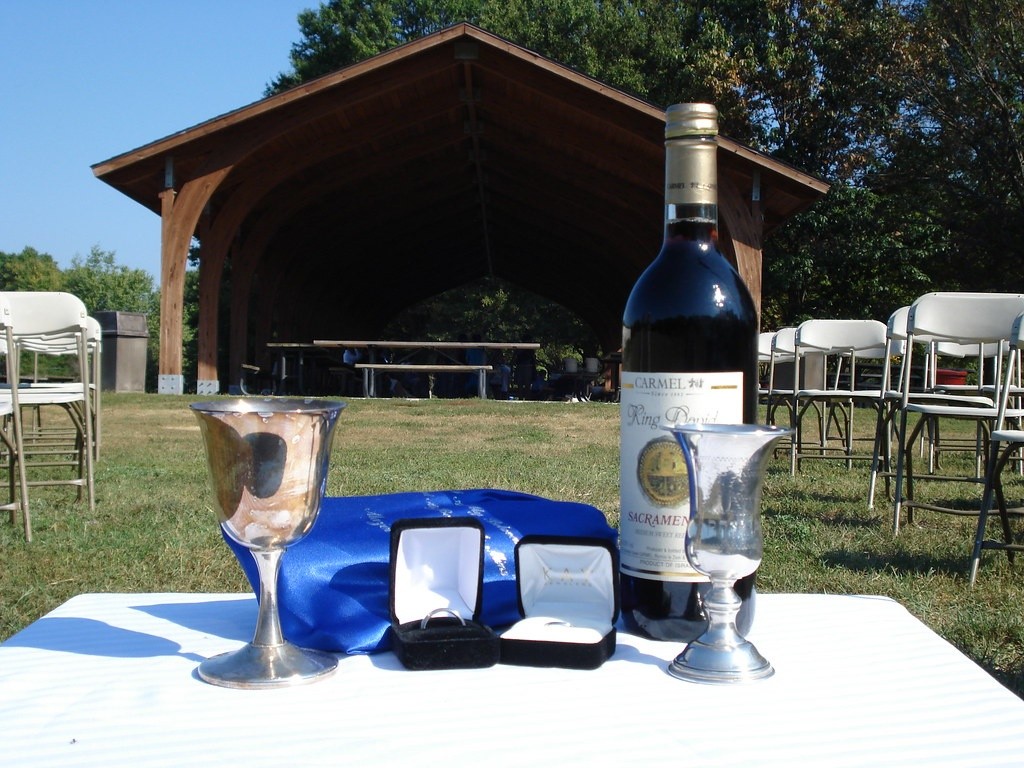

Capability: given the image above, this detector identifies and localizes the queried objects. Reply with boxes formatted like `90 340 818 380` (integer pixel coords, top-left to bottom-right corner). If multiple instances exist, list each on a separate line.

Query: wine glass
660 423 795 684
189 398 349 689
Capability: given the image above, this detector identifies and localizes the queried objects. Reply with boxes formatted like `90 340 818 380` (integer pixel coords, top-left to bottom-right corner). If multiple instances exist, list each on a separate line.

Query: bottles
619 102 760 647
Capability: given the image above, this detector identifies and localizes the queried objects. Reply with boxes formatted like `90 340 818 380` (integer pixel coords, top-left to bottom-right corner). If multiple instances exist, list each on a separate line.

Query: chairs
0 291 104 543
756 292 1024 589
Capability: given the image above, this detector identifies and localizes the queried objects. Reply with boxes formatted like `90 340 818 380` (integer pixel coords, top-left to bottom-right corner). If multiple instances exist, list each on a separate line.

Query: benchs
354 363 493 400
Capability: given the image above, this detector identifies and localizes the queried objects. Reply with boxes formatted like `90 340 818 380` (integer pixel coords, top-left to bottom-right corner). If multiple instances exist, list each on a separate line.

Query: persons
342 333 536 400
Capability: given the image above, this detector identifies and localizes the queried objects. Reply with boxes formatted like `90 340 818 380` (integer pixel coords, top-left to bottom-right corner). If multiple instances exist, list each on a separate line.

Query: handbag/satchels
218 490 618 656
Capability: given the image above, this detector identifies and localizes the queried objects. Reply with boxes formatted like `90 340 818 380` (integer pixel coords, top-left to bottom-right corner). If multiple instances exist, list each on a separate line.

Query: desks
268 343 313 396
0 593 1023 766
313 339 541 400
